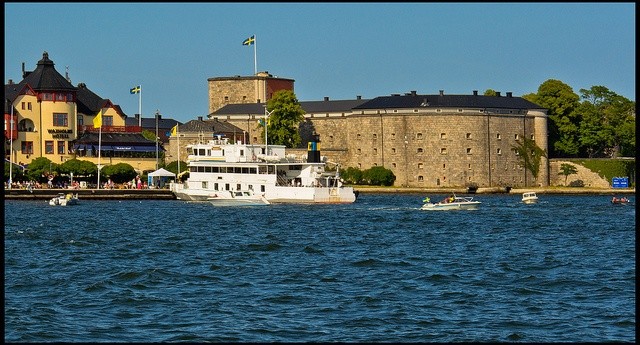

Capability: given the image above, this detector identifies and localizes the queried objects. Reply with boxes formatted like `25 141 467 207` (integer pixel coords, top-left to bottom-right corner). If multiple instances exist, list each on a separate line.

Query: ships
171 143 359 206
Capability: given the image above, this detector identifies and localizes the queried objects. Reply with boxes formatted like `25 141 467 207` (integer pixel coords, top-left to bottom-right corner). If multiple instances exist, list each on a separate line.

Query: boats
49 193 78 207
522 192 538 205
611 199 635 207
418 197 481 210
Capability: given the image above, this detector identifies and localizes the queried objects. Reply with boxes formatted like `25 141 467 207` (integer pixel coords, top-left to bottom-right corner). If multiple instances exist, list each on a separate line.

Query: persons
66 191 73 199
449 195 455 203
612 195 628 202
48 181 79 189
8 180 40 188
104 177 148 189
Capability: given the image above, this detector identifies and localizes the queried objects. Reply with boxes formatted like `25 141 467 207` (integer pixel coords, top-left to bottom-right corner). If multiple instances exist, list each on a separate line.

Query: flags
170 126 176 136
93 111 103 129
243 35 254 45
130 86 140 94
422 197 432 203
